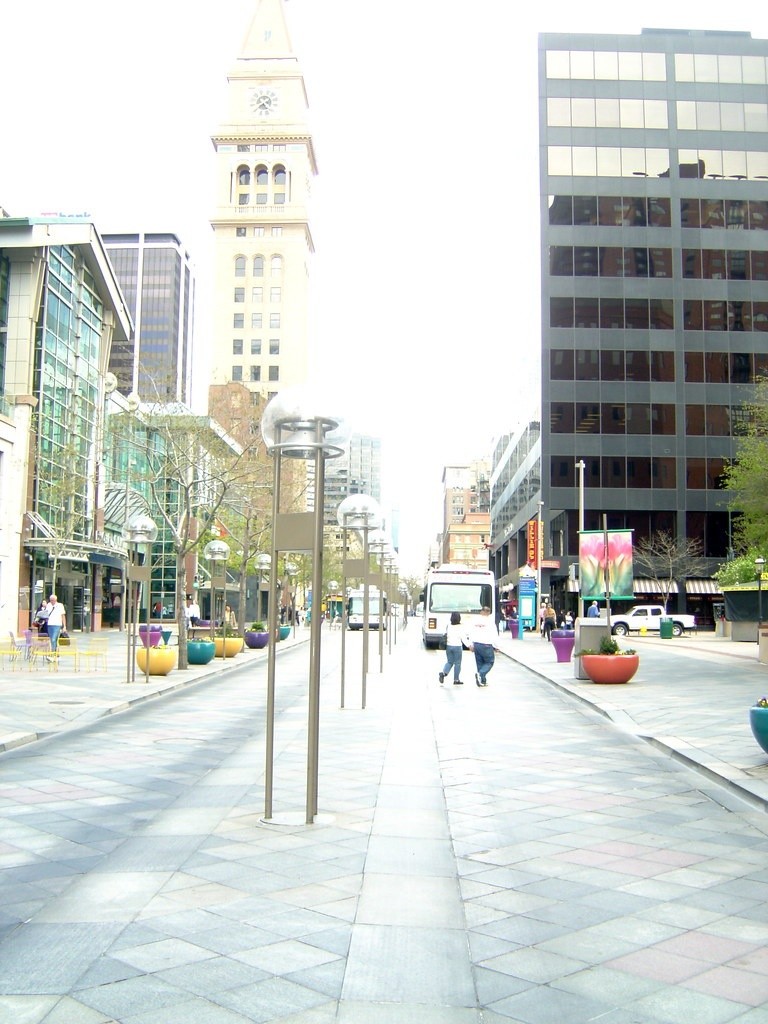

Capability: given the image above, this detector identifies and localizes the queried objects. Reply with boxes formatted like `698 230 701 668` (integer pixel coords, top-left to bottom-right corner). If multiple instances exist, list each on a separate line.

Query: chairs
0 630 110 673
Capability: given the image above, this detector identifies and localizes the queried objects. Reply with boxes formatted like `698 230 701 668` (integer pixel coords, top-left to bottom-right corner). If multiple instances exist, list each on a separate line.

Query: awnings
566 579 730 594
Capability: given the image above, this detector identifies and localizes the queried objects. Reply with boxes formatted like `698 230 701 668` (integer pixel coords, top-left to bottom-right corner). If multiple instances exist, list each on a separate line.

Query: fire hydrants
640 625 647 637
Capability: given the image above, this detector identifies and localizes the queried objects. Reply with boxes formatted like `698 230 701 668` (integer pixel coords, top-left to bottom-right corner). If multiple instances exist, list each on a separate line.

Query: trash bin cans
575 617 611 681
660 617 673 639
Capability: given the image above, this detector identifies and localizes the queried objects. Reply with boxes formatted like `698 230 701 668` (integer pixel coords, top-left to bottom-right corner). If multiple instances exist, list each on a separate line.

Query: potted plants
187 637 216 665
207 616 244 657
574 635 640 683
749 696 768 755
277 624 291 640
243 621 271 650
136 644 176 676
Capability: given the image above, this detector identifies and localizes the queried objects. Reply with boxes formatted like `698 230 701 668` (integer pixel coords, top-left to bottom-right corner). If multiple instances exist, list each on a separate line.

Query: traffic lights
198 575 205 588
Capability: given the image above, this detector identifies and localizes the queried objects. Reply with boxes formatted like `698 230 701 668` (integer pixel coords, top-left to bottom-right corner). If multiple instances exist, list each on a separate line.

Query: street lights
254 553 272 624
337 494 382 710
536 499 544 633
260 386 348 825
361 525 398 675
285 561 299 625
754 555 765 645
327 581 340 625
203 539 230 661
574 460 585 617
120 514 158 684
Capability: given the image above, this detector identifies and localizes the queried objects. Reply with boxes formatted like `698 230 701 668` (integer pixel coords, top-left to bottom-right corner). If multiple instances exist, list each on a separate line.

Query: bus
415 602 424 617
346 589 388 631
419 562 497 648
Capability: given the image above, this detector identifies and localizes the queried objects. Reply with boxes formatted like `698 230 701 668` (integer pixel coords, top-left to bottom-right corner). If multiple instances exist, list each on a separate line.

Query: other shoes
454 680 463 684
439 673 444 682
475 673 488 687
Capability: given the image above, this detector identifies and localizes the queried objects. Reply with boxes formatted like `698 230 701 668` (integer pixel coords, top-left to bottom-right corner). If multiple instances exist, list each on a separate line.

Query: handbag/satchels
59 633 70 645
40 621 47 633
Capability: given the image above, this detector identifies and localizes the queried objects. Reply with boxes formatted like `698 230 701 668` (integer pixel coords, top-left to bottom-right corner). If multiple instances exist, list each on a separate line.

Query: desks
15 641 40 660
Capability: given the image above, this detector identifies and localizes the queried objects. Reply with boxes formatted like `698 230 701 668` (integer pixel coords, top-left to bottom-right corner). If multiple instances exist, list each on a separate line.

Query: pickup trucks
610 605 695 637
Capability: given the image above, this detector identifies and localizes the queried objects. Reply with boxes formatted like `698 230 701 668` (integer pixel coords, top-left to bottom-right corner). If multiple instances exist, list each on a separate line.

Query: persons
281 604 288 625
185 597 200 630
297 601 326 627
540 601 557 641
334 606 339 617
586 600 600 617
470 606 501 686
153 600 161 619
637 610 647 615
439 612 473 685
223 604 236 628
35 594 68 663
500 604 519 632
559 610 574 630
